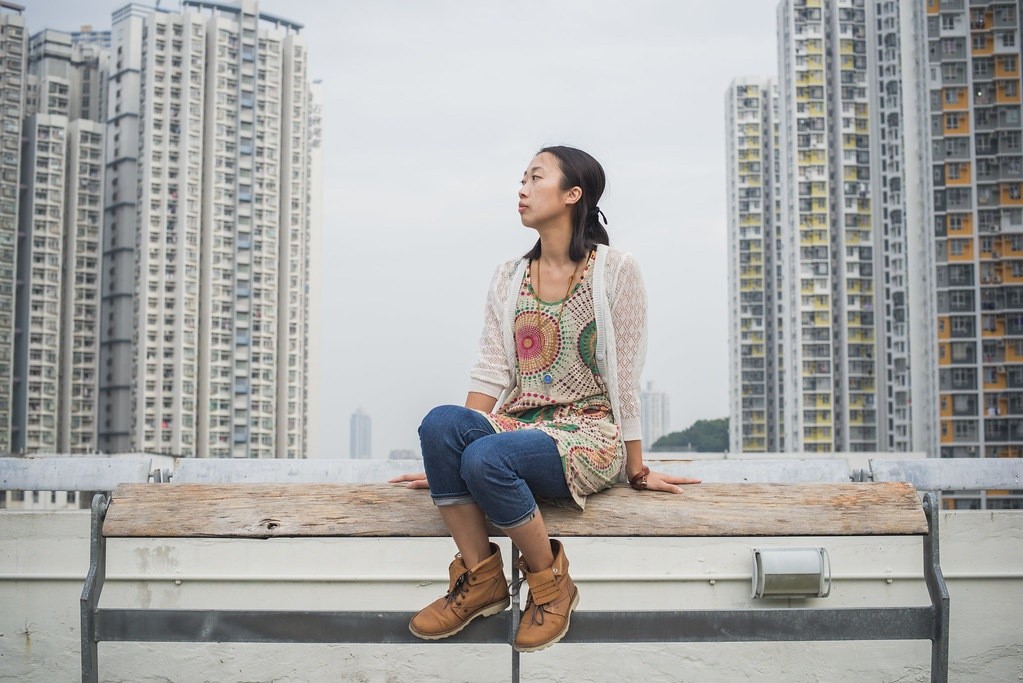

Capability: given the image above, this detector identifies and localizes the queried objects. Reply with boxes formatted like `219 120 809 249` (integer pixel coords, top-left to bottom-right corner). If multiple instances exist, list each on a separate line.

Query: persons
387 146 702 653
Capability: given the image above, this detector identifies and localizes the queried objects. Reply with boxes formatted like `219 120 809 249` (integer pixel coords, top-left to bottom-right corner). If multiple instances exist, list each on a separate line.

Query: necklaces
537 257 579 384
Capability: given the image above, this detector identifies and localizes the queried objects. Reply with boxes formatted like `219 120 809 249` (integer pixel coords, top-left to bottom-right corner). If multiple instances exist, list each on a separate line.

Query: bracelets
629 466 650 490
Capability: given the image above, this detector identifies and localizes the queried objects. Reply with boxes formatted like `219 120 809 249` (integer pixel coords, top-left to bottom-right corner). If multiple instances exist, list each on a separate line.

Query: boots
506 539 580 652
409 542 511 640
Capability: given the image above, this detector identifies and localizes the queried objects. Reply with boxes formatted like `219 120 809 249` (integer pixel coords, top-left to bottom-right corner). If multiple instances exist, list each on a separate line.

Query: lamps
749 547 831 600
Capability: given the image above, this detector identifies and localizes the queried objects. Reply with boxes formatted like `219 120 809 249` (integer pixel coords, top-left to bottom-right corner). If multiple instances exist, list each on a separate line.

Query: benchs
80 480 950 683
